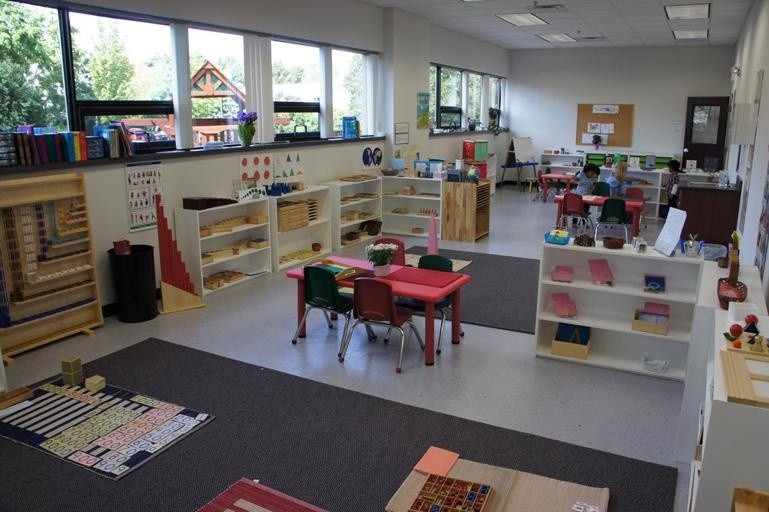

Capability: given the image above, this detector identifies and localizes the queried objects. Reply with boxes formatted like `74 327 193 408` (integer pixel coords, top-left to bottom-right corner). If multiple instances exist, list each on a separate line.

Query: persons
571 161 601 230
663 159 681 210
605 159 639 225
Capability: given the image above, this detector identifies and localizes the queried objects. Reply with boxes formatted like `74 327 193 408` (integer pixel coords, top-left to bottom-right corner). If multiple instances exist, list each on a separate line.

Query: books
0 120 135 166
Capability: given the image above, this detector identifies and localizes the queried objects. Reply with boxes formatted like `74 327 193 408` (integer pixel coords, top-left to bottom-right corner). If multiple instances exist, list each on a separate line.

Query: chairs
284 237 472 375
553 182 645 238
524 169 541 193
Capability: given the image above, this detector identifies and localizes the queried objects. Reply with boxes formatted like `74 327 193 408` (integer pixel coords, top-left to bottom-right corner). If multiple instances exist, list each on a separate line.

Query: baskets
717 277 747 310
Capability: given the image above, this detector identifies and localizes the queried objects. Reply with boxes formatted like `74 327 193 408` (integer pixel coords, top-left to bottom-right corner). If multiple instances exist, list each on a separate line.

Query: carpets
404 244 541 337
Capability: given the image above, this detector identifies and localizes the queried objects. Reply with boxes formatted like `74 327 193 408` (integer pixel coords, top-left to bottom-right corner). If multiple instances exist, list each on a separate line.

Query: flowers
234 109 258 124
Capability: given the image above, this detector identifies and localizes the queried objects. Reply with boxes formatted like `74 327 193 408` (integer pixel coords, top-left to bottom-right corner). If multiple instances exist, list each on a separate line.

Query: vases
237 124 256 145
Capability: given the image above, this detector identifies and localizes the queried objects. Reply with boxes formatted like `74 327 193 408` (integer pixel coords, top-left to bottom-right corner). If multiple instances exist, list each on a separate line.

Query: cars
128 126 170 142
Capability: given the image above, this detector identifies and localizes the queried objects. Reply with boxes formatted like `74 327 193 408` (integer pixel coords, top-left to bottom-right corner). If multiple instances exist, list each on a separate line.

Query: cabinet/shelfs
597 165 741 248
531 235 704 386
382 173 492 244
686 259 768 512
175 175 382 299
540 153 586 177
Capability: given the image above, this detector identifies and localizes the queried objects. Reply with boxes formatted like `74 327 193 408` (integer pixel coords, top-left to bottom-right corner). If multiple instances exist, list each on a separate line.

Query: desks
539 174 576 204
